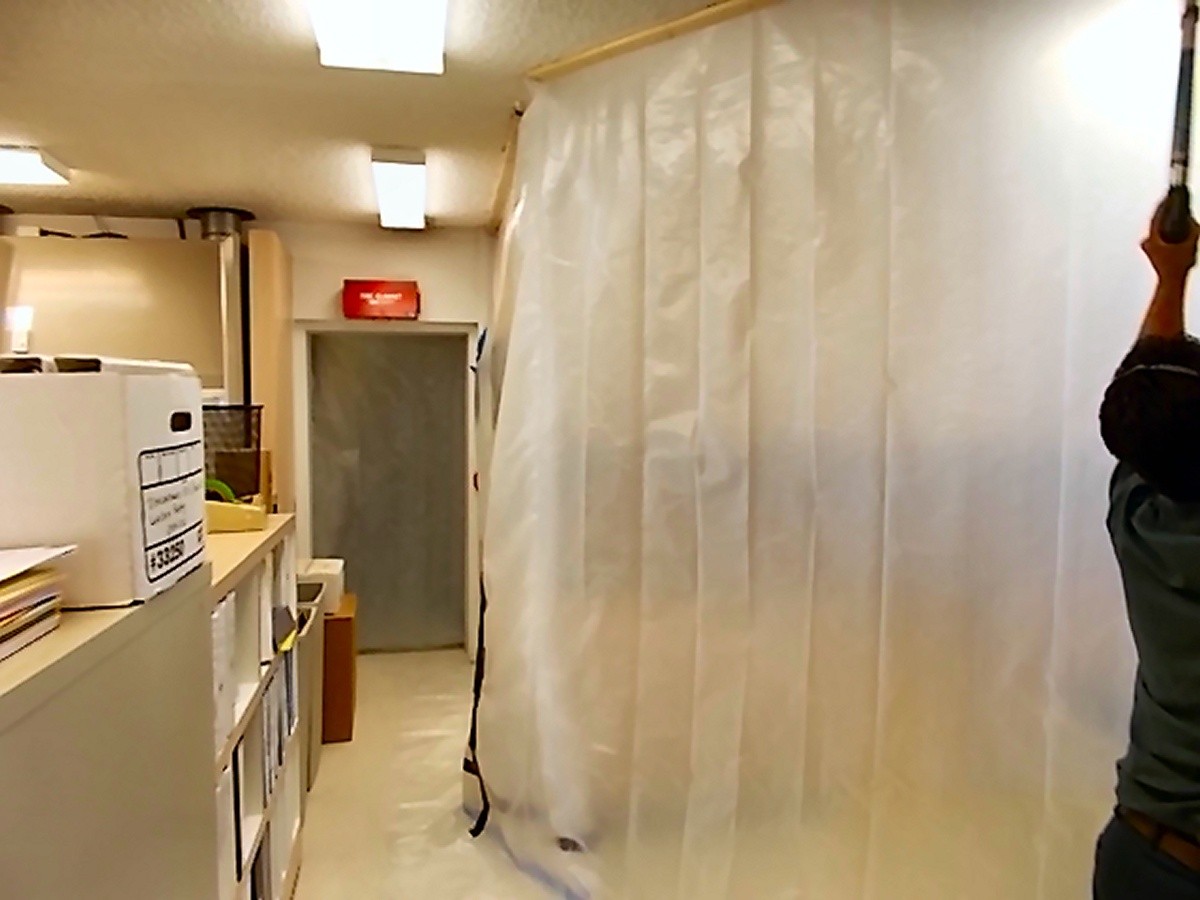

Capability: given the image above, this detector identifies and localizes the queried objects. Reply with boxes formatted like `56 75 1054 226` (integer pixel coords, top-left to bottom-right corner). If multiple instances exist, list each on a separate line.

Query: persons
1089 194 1200 900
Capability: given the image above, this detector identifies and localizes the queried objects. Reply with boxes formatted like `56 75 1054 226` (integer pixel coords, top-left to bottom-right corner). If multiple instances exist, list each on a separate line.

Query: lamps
1 0 446 231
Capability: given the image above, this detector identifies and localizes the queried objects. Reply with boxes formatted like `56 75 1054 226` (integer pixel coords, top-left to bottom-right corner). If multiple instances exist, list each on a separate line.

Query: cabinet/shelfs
0 510 305 900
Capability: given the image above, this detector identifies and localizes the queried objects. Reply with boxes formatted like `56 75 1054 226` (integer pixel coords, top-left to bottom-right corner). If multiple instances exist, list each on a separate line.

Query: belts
1118 803 1200 872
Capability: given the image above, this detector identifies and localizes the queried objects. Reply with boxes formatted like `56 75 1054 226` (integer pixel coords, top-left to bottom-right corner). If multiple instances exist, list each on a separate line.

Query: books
0 538 78 668
